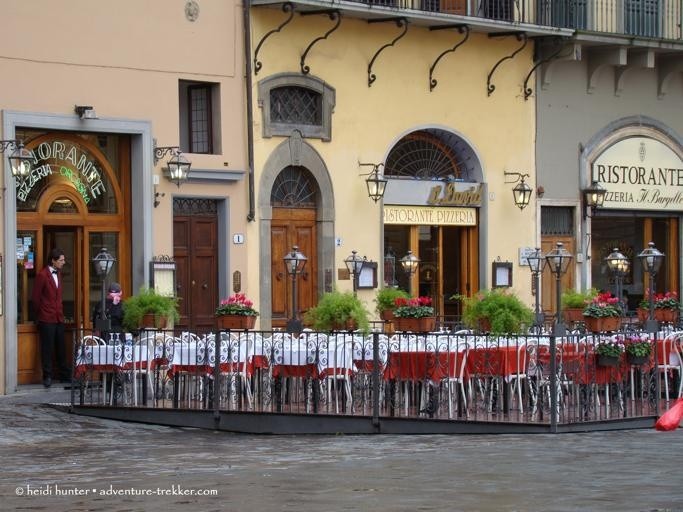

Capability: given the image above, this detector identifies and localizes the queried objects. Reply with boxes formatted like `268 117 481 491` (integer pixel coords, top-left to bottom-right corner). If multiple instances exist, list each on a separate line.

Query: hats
108 283 121 293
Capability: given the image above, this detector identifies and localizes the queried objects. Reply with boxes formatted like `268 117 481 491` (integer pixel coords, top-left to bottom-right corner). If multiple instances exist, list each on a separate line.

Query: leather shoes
43 376 78 388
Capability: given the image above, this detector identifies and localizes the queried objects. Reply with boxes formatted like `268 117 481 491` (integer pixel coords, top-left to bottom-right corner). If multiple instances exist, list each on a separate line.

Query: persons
33 247 68 389
94 283 127 345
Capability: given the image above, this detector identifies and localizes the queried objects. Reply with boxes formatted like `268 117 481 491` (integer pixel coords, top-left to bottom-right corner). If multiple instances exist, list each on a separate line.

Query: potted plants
462 291 534 335
300 292 371 336
375 289 412 320
562 288 600 323
122 284 183 333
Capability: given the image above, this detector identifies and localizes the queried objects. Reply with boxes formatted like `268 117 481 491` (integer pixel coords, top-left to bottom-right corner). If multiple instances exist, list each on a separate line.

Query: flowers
582 292 624 318
214 294 258 316
392 295 434 319
638 288 682 313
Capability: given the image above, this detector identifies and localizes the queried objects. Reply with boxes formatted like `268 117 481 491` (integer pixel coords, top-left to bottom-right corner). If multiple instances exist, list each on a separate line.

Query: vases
393 317 435 332
638 309 679 323
587 316 622 332
214 317 255 328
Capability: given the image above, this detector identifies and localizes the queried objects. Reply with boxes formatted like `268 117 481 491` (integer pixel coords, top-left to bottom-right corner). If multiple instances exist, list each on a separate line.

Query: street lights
283 243 307 331
398 250 420 297
604 242 665 330
527 241 573 330
344 249 365 303
92 248 115 331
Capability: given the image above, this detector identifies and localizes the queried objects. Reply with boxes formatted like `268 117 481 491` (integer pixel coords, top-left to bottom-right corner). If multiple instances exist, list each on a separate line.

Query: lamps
1 138 34 183
357 160 386 203
579 180 606 220
506 170 533 212
155 144 191 188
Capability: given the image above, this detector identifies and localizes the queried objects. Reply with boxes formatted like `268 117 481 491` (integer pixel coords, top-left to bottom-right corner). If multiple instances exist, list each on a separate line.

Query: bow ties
51 270 59 275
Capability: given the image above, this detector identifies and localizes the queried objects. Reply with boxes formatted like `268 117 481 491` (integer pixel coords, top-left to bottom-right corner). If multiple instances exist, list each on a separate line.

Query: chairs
81 329 682 420
